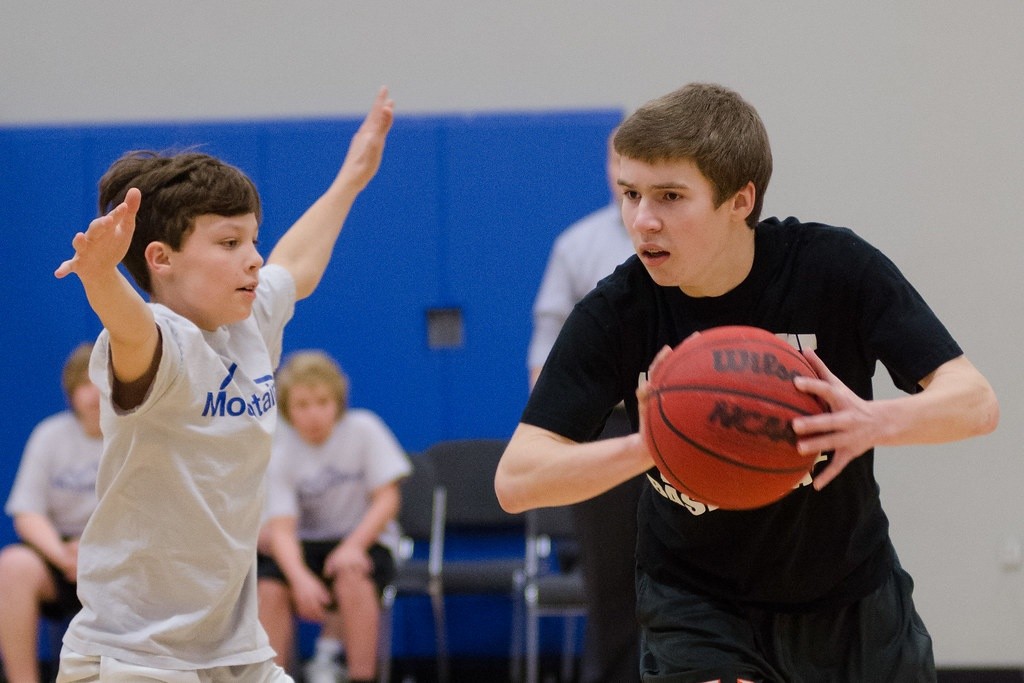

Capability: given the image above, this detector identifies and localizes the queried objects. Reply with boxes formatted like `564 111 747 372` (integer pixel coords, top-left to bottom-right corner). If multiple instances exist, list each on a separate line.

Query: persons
54 84 396 683
530 124 638 683
0 344 106 683
254 349 415 683
493 82 1000 683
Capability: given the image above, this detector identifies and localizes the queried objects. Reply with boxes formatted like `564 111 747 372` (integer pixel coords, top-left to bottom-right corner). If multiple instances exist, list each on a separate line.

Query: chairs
372 437 595 683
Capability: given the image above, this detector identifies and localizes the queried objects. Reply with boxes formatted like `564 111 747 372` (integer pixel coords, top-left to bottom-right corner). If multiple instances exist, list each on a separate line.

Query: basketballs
642 325 830 510
